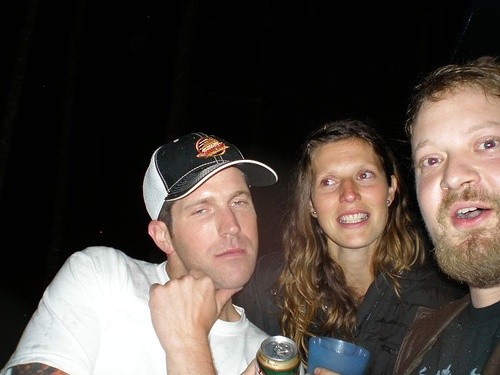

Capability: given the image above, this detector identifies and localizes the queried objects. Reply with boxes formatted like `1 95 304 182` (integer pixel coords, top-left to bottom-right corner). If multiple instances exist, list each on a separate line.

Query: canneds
256 335 301 375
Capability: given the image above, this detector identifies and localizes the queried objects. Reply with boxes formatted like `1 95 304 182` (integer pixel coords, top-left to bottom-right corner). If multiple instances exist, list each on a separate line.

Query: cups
306 336 372 374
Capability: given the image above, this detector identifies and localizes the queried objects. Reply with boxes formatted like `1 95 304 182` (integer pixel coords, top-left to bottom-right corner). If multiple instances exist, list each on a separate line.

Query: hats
143 133 278 222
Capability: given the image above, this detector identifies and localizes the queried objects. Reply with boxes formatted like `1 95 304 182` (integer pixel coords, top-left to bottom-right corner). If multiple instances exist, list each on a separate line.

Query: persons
394 57 499 375
233 122 462 375
0 130 278 375
